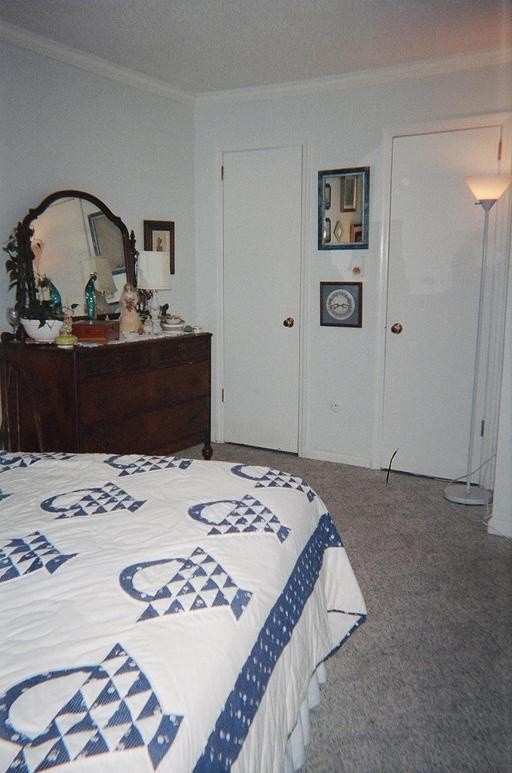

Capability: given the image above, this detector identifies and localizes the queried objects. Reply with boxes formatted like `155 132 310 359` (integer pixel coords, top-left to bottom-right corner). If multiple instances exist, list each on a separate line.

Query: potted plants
4 223 79 342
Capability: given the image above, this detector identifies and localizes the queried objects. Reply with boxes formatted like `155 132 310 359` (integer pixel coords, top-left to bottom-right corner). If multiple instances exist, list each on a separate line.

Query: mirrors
15 190 139 323
317 167 369 249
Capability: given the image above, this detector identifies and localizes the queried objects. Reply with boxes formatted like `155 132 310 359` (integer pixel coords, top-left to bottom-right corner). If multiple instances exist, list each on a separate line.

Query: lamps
443 174 512 506
137 250 171 335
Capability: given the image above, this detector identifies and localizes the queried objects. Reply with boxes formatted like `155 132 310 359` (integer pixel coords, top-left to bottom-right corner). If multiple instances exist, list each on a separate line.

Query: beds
0 451 367 773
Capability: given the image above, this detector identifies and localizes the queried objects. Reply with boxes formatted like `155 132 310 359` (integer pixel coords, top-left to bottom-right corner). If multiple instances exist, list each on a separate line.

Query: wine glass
6 308 21 343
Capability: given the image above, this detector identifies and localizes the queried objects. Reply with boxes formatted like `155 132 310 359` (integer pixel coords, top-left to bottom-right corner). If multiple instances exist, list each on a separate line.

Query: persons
115 282 142 332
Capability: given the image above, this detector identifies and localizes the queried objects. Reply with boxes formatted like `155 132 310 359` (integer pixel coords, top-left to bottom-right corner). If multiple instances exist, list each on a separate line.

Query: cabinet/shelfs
2 332 212 460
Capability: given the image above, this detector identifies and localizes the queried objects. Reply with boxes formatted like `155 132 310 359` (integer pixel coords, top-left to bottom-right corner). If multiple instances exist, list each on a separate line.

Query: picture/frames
143 220 175 275
351 224 362 242
87 210 126 275
341 176 357 210
326 184 331 209
326 219 331 242
320 282 362 328
334 221 344 241
